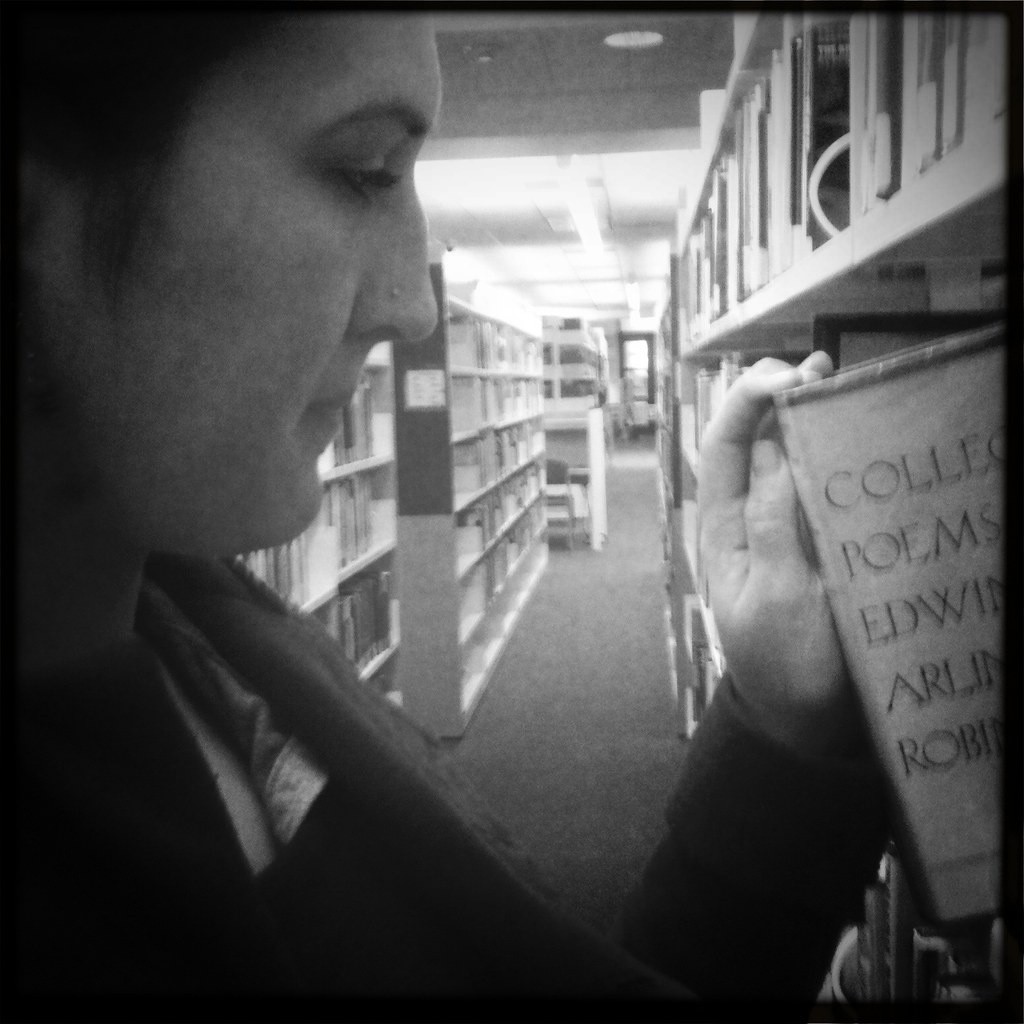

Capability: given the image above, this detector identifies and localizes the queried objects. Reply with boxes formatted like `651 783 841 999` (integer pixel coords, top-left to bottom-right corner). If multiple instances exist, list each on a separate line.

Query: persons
25 13 895 996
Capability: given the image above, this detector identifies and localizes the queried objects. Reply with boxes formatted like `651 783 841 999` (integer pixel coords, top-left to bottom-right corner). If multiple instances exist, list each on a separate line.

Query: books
238 18 1009 682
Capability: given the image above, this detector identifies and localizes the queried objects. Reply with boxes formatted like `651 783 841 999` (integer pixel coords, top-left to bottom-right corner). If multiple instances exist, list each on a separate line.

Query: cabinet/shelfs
234 14 1009 1002
